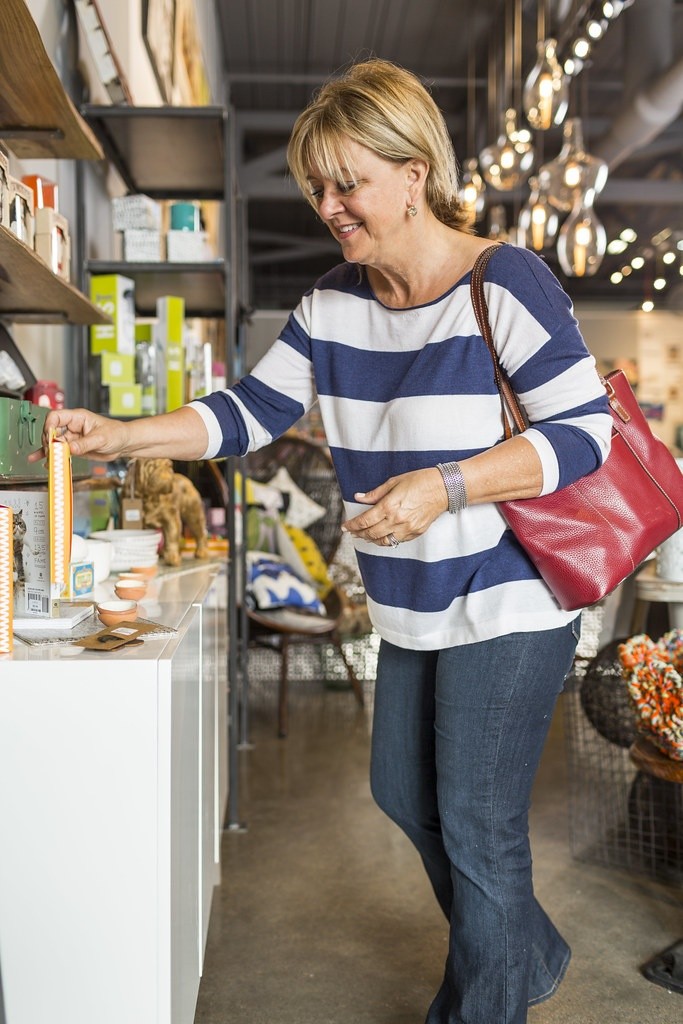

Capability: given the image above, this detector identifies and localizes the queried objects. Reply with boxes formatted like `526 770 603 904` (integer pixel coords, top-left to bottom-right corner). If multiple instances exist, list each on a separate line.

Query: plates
89 528 162 572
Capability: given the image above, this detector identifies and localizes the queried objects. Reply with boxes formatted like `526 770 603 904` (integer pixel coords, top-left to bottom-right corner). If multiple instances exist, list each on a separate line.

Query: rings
388 534 399 548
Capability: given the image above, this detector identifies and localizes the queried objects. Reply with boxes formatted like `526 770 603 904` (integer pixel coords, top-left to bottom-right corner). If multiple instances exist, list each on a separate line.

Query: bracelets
438 462 467 514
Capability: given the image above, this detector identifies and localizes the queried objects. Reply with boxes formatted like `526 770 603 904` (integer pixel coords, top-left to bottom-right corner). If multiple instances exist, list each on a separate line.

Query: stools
248 608 365 738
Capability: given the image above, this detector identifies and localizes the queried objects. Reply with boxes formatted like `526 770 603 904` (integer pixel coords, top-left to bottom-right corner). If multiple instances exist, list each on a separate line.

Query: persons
26 53 612 1023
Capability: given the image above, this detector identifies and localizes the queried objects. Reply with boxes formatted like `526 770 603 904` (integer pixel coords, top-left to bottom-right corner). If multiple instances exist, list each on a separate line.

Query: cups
129 563 159 579
97 600 139 627
117 573 150 584
113 581 148 601
170 204 199 233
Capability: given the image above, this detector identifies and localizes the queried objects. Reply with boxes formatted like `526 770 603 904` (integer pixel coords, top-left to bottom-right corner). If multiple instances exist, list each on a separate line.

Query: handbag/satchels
471 244 683 612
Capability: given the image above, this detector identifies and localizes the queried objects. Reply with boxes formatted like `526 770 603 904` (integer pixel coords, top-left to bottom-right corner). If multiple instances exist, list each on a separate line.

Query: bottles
33 380 59 409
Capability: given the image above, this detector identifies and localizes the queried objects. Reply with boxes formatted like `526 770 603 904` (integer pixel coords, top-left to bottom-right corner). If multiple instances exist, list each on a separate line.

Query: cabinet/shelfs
0 0 241 1022
